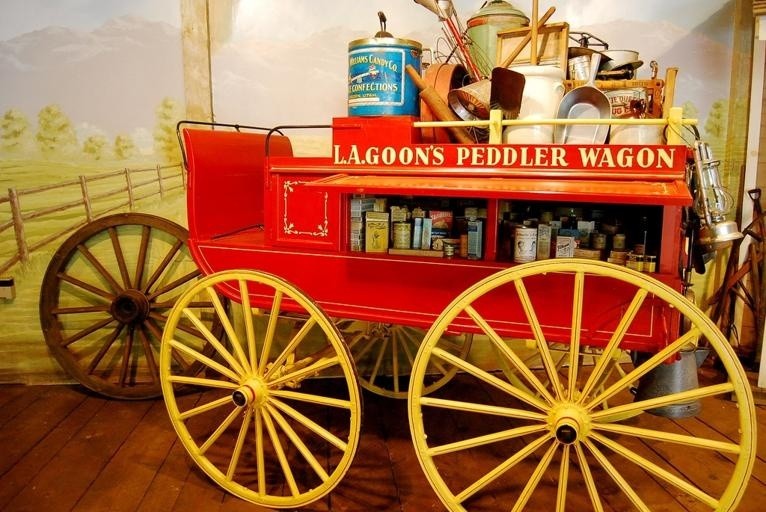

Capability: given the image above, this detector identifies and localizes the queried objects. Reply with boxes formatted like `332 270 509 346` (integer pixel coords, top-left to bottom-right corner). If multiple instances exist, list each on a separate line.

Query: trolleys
157 121 756 512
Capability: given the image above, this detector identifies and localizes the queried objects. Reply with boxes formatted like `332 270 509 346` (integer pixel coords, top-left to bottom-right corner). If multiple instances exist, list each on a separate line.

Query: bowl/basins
599 50 639 80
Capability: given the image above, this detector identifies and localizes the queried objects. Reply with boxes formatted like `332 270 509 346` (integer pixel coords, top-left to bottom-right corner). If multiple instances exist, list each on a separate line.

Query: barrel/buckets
503 66 566 145
348 38 421 116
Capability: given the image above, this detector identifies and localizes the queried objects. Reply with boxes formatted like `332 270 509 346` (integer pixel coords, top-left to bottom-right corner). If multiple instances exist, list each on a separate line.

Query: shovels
553 53 612 144
